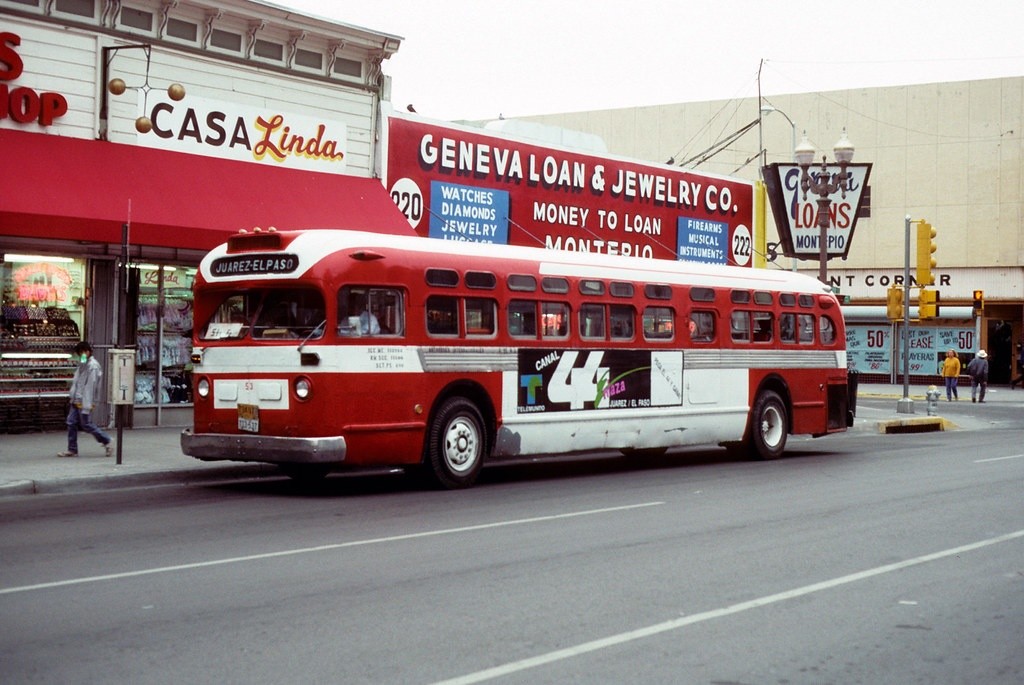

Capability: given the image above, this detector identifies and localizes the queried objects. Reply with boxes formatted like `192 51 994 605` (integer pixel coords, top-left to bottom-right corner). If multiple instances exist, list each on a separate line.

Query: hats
977 350 987 358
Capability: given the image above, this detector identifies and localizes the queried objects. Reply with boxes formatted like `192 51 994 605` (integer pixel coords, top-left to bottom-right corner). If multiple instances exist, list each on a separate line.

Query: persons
522 312 536 334
337 290 381 337
966 350 988 404
56 342 115 457
942 349 961 402
1011 350 1024 389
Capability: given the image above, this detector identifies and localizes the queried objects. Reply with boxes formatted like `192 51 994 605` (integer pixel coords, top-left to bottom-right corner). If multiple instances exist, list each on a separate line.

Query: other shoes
947 399 951 401
979 401 984 402
104 438 115 456
973 398 975 402
955 397 958 401
1010 380 1015 389
57 450 79 457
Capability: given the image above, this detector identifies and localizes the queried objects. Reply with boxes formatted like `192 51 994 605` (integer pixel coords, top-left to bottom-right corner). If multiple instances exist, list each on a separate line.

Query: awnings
0 128 420 251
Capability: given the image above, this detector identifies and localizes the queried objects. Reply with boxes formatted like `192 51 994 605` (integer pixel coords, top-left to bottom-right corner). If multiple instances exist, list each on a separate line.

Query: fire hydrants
924 385 942 417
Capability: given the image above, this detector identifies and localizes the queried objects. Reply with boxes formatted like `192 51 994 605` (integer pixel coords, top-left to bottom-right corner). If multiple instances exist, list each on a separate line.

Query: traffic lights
974 290 985 309
915 223 939 286
918 289 941 319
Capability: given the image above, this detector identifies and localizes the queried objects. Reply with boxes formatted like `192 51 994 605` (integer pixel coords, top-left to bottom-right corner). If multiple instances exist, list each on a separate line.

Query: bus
178 226 858 488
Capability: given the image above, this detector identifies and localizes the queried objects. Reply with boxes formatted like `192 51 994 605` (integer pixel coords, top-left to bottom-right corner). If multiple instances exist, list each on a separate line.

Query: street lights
760 105 798 163
793 127 856 284
